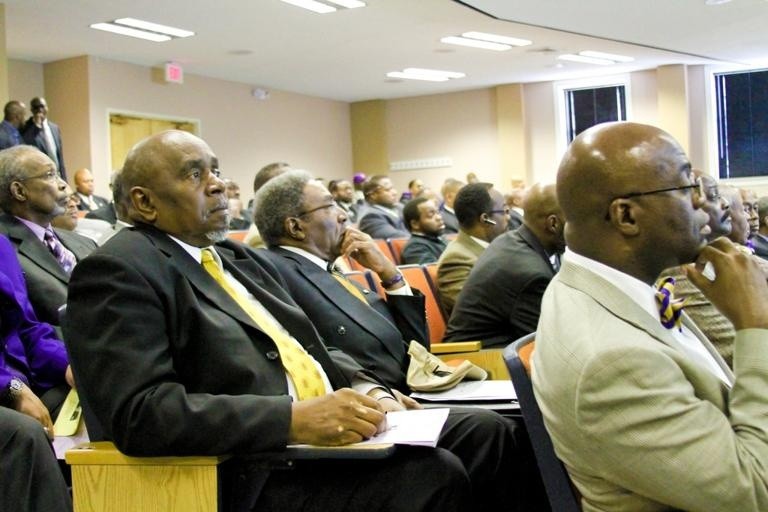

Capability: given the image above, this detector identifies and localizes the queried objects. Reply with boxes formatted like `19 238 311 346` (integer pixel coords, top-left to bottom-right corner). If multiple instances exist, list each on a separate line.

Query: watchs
380 269 405 289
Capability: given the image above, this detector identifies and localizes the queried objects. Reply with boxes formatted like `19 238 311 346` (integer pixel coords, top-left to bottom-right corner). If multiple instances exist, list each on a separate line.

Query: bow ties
655 276 686 329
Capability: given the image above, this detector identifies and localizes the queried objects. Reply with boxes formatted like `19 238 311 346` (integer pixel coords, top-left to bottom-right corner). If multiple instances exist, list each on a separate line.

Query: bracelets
10 377 23 403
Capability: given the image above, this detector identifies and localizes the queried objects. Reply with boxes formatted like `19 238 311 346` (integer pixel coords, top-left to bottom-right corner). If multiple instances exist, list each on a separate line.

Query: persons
441 183 566 348
73 167 108 212
399 198 448 264
84 168 121 225
50 180 80 231
505 186 529 231
61 129 516 512
0 233 77 442
438 180 465 234
221 177 255 230
528 120 768 512
434 182 512 321
328 177 358 224
726 184 768 258
250 167 430 409
0 99 28 150
354 172 368 203
401 178 430 206
0 144 100 344
22 96 69 183
358 175 410 240
654 167 738 373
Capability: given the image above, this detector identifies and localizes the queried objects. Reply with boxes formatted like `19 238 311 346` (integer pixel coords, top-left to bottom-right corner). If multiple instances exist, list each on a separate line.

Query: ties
44 228 78 276
200 248 327 401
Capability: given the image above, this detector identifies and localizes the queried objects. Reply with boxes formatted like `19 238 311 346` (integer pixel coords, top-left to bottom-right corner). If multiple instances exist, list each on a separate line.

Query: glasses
603 176 704 221
489 208 510 215
20 170 62 182
298 201 338 218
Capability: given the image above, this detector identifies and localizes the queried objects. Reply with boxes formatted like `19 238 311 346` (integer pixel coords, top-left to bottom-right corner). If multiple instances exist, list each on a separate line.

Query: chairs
501 330 590 511
391 235 421 261
56 304 292 511
424 258 475 323
345 269 482 381
366 263 512 378
345 236 396 281
